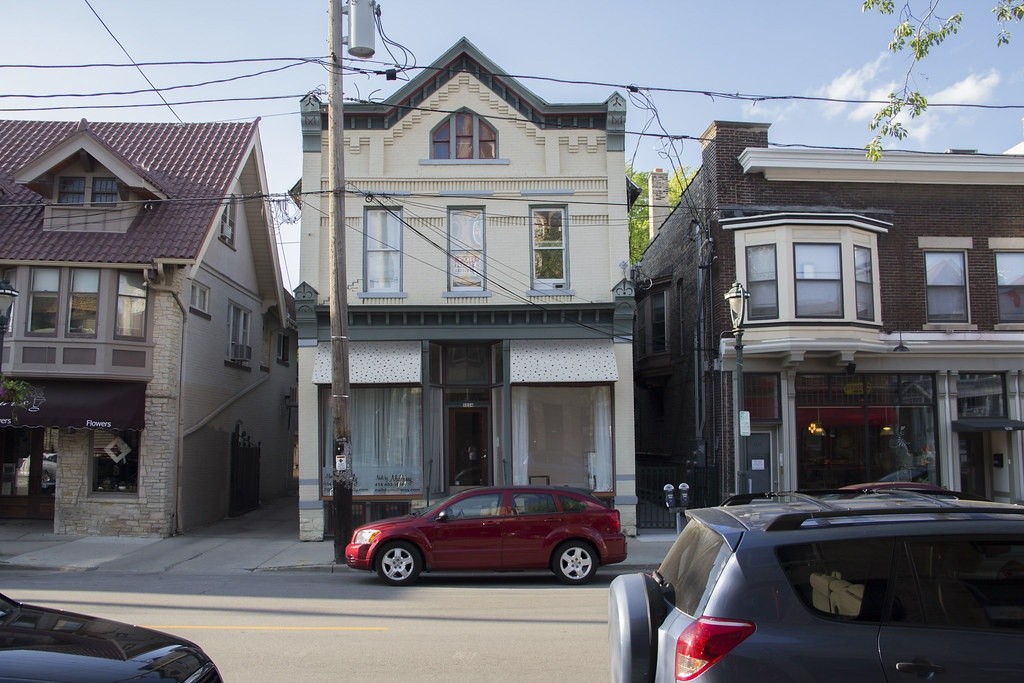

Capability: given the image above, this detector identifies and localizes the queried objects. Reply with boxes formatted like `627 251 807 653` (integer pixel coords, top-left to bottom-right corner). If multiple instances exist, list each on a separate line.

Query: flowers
0 377 34 409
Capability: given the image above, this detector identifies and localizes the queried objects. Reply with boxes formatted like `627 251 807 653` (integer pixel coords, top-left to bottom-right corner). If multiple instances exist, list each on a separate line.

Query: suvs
608 489 1023 683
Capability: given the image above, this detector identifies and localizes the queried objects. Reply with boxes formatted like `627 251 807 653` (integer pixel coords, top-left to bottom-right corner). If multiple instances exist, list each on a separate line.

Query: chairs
940 560 991 626
808 570 863 619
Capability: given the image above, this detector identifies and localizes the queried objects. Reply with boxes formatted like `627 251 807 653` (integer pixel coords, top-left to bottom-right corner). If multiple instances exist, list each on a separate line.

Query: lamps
808 408 826 435
880 408 895 435
887 327 909 352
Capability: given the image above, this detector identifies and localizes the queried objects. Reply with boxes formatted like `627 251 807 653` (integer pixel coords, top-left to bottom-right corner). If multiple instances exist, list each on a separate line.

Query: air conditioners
231 344 251 362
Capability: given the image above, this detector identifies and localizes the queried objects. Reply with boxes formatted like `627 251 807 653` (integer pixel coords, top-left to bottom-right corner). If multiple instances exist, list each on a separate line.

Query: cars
23 453 57 487
0 591 223 683
345 484 632 586
